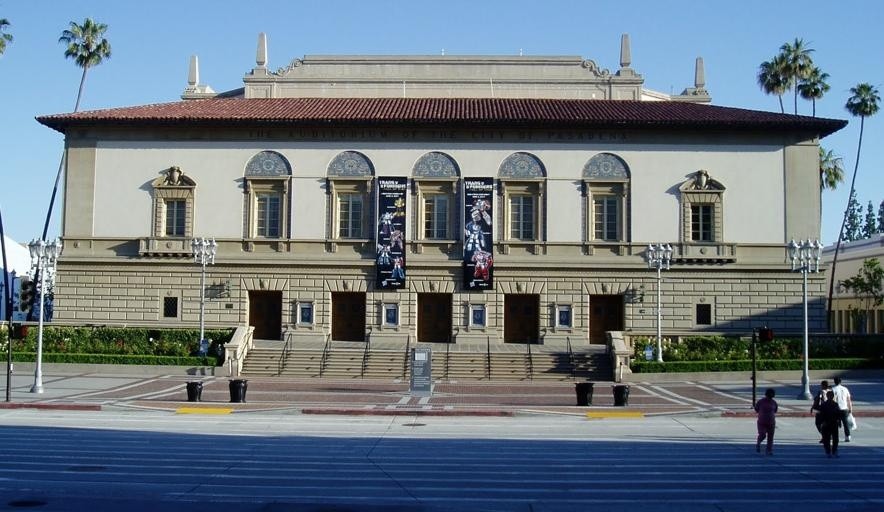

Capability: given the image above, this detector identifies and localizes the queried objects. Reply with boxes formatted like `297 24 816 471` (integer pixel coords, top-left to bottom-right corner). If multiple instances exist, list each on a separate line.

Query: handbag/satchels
812 397 824 411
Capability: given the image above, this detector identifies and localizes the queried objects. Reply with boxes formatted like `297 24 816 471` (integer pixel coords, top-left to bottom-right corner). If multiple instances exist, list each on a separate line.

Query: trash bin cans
613 384 629 406
228 380 248 403
186 380 203 402
575 383 595 406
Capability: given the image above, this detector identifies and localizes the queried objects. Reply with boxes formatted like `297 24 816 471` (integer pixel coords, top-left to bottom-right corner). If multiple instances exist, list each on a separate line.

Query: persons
814 380 832 443
819 391 841 459
754 389 778 455
831 376 852 442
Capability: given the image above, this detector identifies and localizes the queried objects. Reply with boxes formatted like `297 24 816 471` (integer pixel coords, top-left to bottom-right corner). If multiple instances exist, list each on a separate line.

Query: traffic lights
20 276 32 314
14 326 27 339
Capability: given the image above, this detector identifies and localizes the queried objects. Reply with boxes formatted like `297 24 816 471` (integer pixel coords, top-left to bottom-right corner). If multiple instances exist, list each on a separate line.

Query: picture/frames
552 301 575 333
295 298 316 330
467 300 487 333
380 300 401 331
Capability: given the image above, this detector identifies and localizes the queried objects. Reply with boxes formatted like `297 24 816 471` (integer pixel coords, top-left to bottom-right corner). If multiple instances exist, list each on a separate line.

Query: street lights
786 240 824 401
28 238 63 393
646 244 672 362
193 237 218 352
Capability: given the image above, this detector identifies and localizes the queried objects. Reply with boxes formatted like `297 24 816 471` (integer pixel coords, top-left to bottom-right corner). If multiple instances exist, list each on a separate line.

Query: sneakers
845 436 851 442
